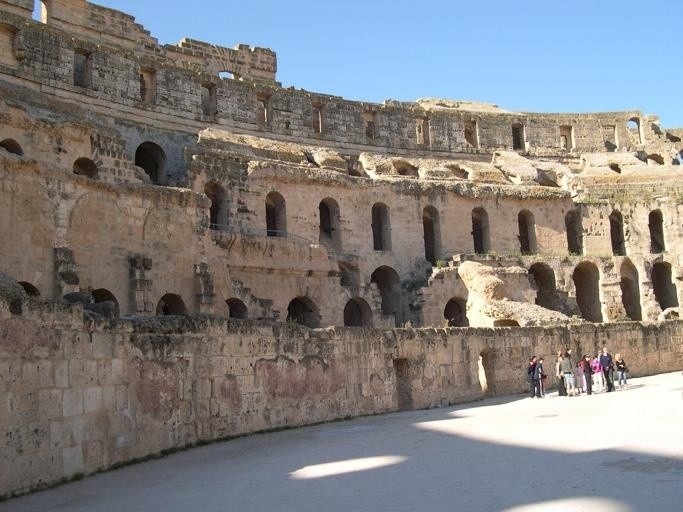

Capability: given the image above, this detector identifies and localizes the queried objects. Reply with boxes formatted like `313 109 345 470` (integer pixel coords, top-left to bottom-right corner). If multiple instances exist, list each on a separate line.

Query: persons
615 353 627 387
555 345 615 396
532 358 546 398
526 356 538 397
